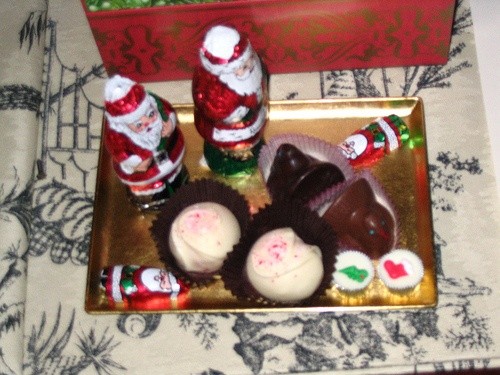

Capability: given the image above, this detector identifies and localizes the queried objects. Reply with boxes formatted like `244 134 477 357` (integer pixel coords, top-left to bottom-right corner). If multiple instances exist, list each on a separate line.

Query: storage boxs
76 0 457 88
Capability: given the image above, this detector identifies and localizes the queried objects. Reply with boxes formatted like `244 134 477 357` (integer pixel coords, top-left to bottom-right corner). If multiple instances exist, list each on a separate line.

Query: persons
338 113 409 168
103 74 189 210
192 26 270 174
100 264 191 301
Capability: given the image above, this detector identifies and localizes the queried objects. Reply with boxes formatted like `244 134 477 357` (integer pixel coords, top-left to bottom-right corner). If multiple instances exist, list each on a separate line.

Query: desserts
169 142 425 306
